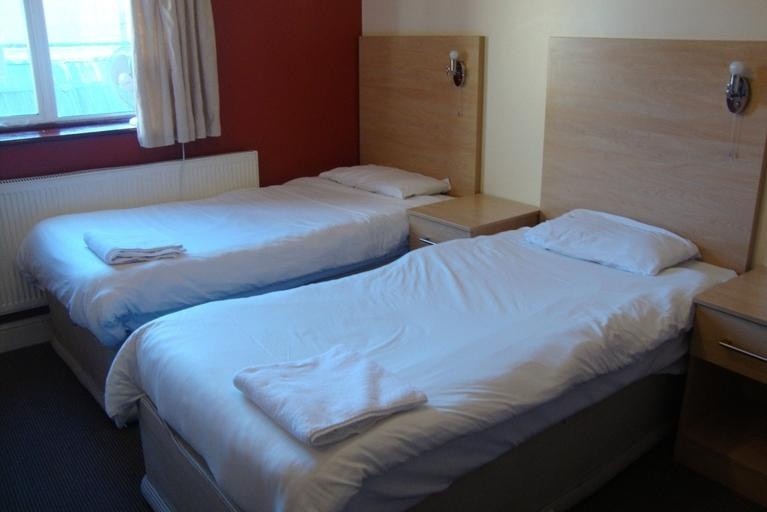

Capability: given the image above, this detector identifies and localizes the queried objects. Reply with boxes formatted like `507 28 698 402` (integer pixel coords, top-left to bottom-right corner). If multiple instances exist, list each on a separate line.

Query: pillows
317 164 451 200
521 208 702 275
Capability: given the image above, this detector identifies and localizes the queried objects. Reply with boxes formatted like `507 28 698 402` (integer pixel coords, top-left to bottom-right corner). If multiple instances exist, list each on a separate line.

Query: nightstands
406 193 539 252
671 265 765 508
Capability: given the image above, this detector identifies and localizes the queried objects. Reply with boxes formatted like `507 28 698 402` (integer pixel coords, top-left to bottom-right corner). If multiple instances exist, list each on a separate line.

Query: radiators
0 149 259 319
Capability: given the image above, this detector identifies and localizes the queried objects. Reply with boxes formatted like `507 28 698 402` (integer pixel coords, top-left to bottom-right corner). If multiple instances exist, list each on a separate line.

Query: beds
134 228 740 511
30 175 458 423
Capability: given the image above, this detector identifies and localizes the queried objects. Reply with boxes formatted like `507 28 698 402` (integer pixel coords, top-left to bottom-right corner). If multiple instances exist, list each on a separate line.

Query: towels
82 225 187 265
231 343 428 448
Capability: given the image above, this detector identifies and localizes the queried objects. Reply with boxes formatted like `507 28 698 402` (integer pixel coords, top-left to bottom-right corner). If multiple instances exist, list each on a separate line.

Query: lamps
723 60 751 114
445 50 465 88
